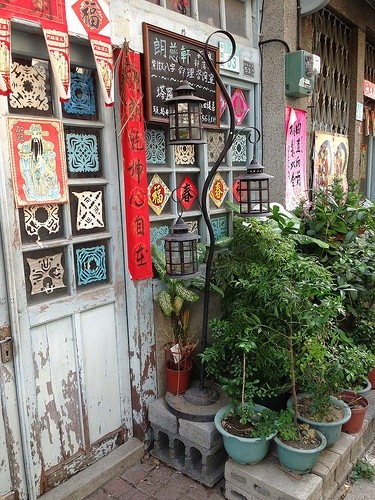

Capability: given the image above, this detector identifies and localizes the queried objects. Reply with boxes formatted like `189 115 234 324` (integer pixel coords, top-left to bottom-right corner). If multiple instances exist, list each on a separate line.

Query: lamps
235 144 273 219
164 64 206 144
161 202 201 280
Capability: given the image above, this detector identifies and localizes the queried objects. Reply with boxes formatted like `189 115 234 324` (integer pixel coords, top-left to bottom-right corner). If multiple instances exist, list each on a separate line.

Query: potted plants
151 177 373 475
